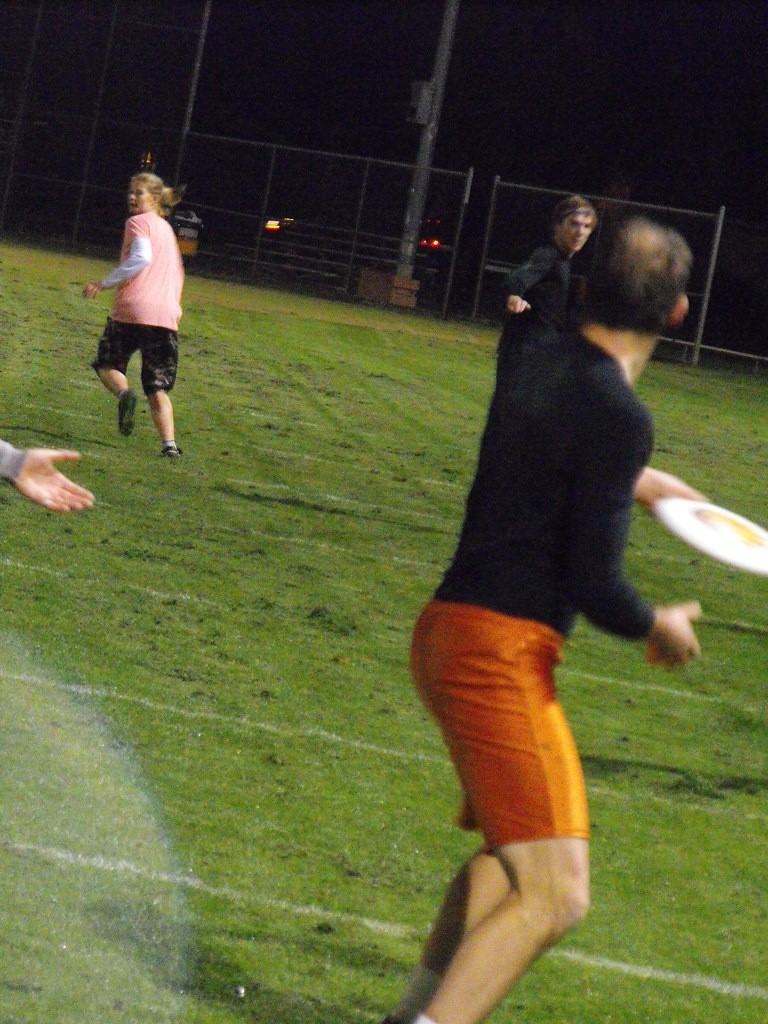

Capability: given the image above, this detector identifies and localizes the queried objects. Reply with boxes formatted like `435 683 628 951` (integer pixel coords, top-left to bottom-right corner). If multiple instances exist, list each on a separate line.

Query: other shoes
160 446 183 458
118 390 137 436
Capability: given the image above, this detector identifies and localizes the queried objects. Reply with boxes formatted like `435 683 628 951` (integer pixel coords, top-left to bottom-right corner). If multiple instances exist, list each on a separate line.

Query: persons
82 172 185 459
0 438 96 513
380 216 710 1024
501 195 599 330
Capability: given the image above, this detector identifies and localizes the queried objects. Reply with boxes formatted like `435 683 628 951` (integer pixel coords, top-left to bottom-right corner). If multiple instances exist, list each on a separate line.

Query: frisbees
654 496 768 577
